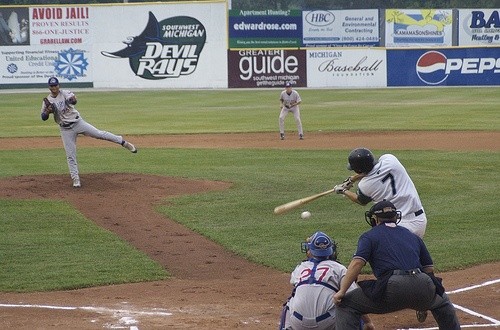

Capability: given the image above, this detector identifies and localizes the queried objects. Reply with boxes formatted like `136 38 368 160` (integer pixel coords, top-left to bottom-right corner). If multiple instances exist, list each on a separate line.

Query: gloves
343 176 354 190
334 184 346 194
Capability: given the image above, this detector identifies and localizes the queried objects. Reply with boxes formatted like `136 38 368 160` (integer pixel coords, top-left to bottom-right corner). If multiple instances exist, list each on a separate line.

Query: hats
285 82 291 87
371 198 397 219
307 231 334 257
48 76 59 86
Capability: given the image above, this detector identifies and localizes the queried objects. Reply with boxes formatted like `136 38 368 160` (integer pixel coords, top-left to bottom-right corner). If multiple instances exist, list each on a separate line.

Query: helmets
347 148 374 173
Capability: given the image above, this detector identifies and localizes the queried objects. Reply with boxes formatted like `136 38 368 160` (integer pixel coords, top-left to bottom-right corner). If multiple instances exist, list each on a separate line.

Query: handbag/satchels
356 272 389 304
425 272 445 298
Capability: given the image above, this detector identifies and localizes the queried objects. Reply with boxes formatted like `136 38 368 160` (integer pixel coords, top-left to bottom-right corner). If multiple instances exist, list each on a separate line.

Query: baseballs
301 211 311 219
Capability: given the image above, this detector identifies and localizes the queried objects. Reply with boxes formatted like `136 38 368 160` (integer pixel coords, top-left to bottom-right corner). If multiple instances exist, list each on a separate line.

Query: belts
392 269 421 275
293 311 331 323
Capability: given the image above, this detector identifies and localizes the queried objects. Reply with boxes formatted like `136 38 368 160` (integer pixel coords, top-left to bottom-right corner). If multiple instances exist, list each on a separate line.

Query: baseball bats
274 188 335 215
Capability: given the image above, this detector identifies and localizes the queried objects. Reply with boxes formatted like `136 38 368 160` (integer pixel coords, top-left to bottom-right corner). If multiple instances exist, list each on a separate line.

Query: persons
332 199 461 330
333 148 427 322
279 231 375 330
40 77 138 188
279 82 304 140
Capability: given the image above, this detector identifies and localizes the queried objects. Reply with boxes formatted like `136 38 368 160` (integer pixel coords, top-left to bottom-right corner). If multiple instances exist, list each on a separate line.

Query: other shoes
279 133 284 139
299 134 303 139
122 140 138 153
73 179 82 189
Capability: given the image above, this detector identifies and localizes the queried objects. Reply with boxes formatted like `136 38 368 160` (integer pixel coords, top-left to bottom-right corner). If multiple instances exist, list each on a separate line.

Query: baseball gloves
44 98 56 113
